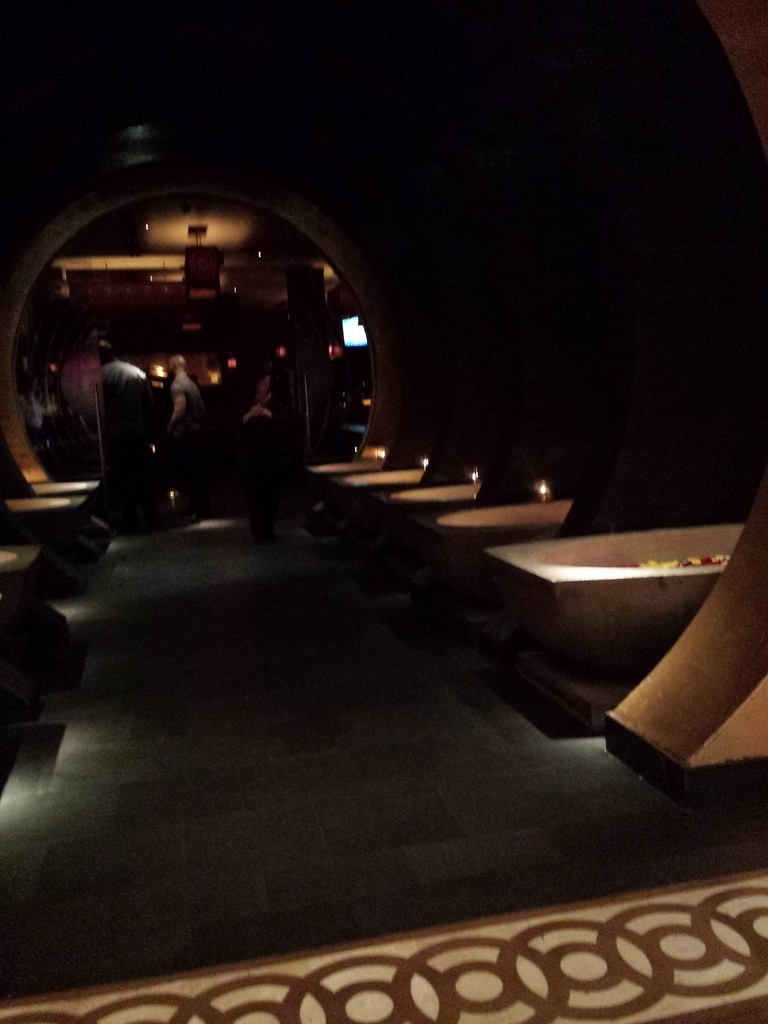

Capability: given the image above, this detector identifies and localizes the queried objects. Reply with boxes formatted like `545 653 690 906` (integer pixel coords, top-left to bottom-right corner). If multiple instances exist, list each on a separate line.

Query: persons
86 338 291 535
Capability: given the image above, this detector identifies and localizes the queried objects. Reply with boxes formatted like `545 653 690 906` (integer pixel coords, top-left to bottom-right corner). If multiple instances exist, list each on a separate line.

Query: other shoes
114 518 139 532
139 514 161 527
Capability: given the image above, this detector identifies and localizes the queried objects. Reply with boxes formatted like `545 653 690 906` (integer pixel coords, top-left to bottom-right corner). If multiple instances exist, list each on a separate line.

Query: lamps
183 225 224 300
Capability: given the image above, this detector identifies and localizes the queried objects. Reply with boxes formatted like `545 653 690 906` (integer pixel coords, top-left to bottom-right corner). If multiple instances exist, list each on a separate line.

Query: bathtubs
309 458 746 655
0 481 100 583
